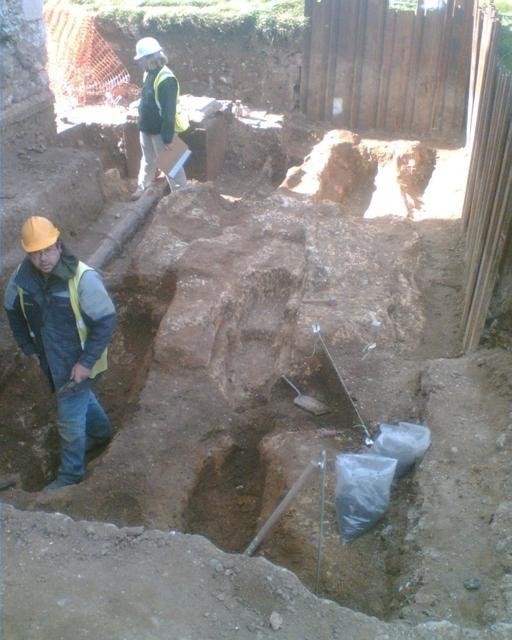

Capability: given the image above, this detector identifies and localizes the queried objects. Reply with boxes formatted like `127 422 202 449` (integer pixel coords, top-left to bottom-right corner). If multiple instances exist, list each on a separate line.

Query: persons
126 37 189 201
3 216 119 493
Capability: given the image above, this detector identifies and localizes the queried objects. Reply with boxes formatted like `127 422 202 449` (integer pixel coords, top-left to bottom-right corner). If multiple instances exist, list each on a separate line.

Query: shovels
281 375 333 416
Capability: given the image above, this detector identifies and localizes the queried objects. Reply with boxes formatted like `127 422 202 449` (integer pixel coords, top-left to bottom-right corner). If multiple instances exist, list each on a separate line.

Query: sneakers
129 187 145 201
41 478 69 493
84 433 109 455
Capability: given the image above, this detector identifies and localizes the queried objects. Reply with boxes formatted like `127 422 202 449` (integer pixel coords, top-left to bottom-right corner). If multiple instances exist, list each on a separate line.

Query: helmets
19 215 62 253
132 36 164 63
